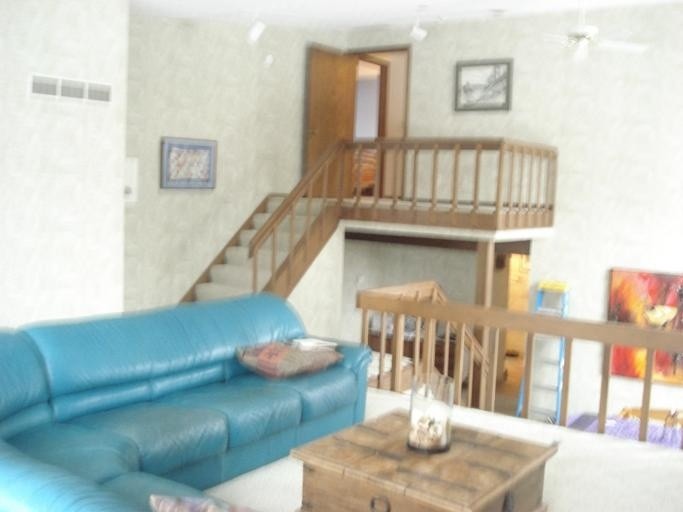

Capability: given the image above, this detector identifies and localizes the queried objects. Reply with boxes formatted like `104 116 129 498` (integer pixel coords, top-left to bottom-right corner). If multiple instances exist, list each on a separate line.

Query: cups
405 372 455 455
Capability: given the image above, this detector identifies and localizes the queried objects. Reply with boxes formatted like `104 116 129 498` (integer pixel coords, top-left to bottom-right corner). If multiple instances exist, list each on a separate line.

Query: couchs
0 291 374 512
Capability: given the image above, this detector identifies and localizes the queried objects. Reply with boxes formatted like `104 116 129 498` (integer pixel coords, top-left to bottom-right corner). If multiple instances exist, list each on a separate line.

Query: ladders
516 282 569 426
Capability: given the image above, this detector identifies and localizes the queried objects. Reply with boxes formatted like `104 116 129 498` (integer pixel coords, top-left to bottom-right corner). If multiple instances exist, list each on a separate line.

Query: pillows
144 490 250 512
233 339 343 380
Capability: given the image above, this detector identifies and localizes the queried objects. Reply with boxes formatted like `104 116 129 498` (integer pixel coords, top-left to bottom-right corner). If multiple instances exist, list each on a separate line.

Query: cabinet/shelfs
367 331 456 378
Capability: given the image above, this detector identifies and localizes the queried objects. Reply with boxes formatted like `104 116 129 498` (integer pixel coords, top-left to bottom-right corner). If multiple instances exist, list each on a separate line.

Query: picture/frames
157 134 217 191
452 57 513 112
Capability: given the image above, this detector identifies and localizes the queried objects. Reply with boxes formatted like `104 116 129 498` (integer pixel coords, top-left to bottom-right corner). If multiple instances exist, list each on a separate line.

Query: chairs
616 406 679 427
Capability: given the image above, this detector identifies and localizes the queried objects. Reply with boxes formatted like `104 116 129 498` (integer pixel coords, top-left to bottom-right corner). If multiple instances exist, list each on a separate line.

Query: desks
564 412 681 452
